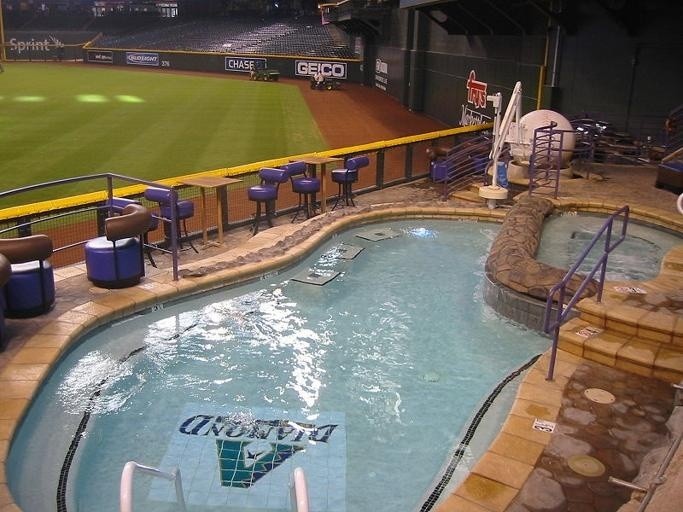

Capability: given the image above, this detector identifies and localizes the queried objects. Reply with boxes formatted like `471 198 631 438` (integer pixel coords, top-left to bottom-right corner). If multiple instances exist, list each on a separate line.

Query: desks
177 176 244 250
289 158 344 214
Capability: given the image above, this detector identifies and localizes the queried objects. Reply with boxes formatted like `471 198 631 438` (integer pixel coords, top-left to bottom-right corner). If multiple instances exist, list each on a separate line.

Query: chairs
107 198 158 268
144 186 200 253
428 147 453 181
331 156 369 211
247 166 288 236
0 234 56 319
0 253 12 351
281 160 321 223
85 203 153 290
